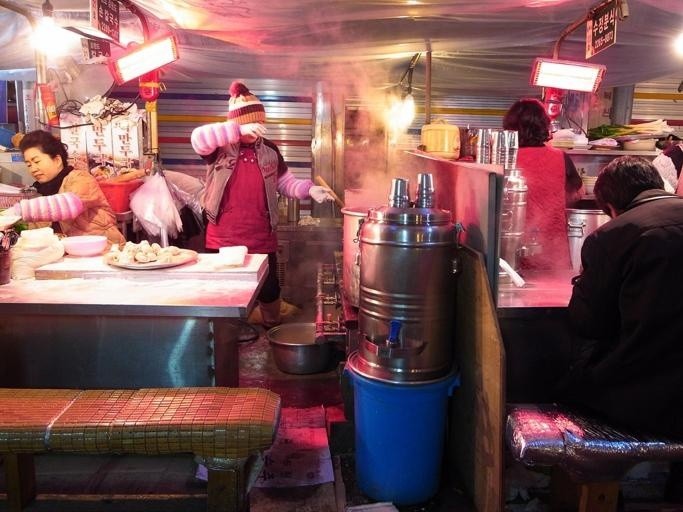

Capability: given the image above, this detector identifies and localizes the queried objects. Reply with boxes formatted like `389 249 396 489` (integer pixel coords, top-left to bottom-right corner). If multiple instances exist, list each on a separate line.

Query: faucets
387 319 403 350
520 238 544 257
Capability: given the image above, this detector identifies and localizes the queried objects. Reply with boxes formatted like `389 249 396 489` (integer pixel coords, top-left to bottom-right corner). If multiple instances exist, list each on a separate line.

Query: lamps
528 57 607 94
109 31 180 87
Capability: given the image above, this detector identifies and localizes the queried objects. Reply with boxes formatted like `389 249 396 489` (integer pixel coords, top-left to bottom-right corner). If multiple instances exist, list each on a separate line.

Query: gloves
237 121 268 140
308 182 337 205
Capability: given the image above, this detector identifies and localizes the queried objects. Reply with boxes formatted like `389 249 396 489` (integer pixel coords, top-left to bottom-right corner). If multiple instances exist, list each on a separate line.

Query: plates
106 248 199 270
550 138 617 150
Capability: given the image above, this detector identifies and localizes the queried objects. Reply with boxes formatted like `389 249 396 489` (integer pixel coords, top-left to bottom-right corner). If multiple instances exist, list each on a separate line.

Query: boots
259 298 282 328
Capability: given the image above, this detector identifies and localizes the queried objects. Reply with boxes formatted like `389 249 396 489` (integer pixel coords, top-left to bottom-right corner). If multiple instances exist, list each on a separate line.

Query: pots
338 203 381 309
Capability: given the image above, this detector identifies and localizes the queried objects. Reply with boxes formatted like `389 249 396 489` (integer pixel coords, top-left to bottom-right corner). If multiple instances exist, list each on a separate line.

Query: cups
389 172 438 207
287 195 301 222
477 127 518 168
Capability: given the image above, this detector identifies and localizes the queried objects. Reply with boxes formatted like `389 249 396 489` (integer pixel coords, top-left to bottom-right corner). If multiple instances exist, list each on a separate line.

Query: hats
226 81 266 125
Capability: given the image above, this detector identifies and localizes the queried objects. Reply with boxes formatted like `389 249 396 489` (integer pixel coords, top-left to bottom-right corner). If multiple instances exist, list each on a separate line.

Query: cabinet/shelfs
565 151 661 200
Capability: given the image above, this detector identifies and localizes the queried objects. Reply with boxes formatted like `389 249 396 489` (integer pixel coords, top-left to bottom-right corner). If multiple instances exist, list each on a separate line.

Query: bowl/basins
624 138 659 151
63 234 107 256
265 322 338 375
583 175 600 196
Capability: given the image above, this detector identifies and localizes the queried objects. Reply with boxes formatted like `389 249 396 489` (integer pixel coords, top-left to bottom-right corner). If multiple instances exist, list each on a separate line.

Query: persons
191 81 336 329
0 130 126 251
503 97 583 271
557 156 683 512
651 140 683 197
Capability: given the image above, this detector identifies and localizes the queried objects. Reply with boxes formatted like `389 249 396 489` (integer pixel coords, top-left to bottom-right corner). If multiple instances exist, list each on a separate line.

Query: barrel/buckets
341 204 370 306
564 207 607 274
358 204 463 383
342 366 463 505
500 170 530 279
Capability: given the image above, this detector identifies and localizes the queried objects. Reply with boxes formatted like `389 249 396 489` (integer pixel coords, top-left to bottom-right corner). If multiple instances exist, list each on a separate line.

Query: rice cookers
421 117 460 160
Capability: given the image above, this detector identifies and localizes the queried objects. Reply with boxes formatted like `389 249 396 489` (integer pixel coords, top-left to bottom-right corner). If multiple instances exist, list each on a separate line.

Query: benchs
505 406 683 512
0 388 281 512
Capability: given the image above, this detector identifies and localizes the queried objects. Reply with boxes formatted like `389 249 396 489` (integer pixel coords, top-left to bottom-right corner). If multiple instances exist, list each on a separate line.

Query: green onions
588 118 674 139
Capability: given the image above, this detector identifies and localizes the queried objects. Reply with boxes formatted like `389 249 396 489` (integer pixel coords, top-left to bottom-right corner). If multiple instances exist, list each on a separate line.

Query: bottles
40 85 59 126
276 193 288 224
